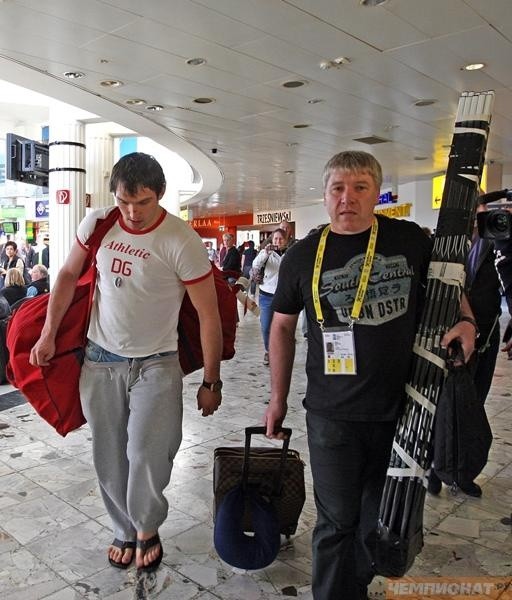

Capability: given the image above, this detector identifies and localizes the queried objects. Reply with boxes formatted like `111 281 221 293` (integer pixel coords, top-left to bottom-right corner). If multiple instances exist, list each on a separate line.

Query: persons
252 229 291 366
27 264 49 298
1 241 25 269
1 268 27 306
206 243 219 265
254 221 297 285
221 234 241 287
29 152 223 573
266 151 478 600
426 188 501 496
238 240 257 301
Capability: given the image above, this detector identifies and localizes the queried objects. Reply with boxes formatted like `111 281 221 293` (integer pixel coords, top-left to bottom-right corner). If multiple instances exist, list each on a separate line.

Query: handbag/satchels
433 346 496 488
249 254 270 285
5 207 239 438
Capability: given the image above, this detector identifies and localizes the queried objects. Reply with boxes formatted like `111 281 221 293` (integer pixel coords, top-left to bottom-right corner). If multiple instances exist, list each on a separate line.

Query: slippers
108 538 135 569
135 530 163 572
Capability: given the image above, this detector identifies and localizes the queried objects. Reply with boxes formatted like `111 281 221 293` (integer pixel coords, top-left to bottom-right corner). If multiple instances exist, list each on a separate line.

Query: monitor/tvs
5 132 49 187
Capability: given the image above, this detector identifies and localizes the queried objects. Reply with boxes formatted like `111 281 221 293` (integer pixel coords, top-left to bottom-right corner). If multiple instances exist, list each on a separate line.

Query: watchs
203 380 224 390
459 316 480 340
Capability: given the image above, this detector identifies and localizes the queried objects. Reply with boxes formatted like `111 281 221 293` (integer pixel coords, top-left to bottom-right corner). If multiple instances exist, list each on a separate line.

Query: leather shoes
428 467 442 495
460 480 482 499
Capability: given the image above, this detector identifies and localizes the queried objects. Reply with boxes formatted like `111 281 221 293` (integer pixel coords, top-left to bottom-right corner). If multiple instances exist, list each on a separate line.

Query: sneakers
263 352 269 365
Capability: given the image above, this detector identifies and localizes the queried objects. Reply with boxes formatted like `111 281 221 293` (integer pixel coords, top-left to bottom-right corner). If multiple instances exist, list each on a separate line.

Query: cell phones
267 245 278 251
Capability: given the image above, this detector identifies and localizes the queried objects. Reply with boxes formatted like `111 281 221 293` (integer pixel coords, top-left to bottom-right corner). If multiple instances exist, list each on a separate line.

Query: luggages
214 427 306 537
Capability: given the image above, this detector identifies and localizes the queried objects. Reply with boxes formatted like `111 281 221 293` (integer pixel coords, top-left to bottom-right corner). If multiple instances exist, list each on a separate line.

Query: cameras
475 189 512 240
